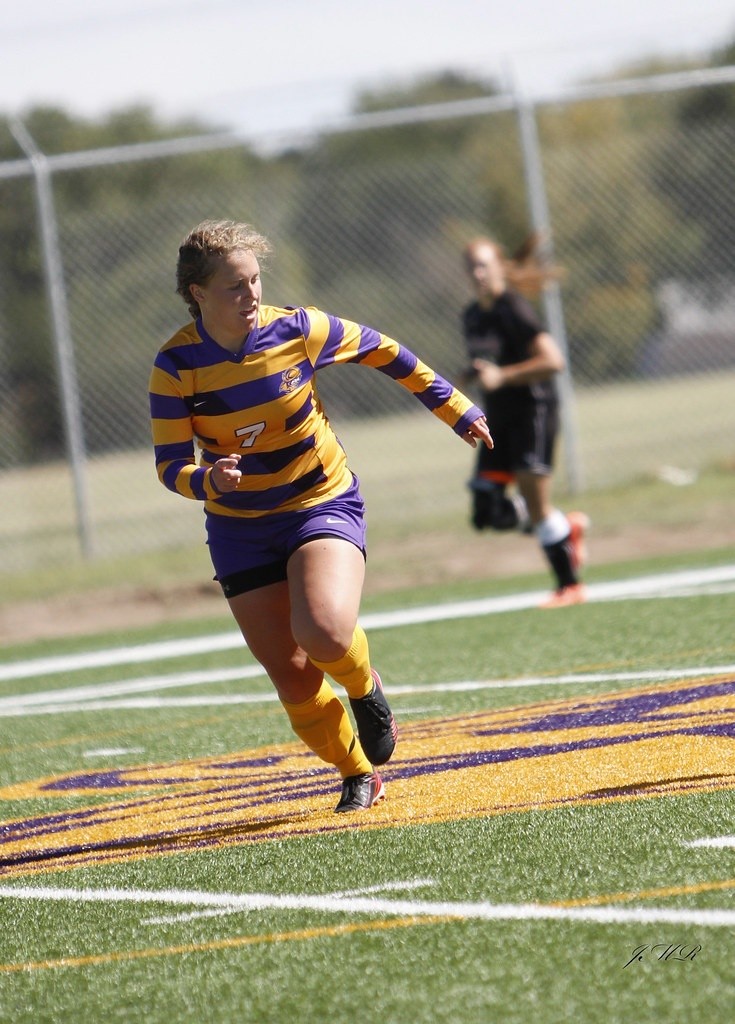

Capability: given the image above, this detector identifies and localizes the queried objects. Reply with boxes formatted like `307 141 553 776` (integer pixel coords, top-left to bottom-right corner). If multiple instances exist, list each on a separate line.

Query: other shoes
563 511 587 570
541 584 584 608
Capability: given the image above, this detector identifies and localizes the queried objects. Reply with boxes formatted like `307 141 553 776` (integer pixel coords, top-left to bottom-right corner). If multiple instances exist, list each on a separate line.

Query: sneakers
334 767 386 813
348 668 398 765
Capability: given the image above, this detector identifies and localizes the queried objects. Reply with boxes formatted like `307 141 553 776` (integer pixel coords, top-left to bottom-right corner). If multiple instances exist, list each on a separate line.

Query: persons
455 235 587 606
150 220 493 813
